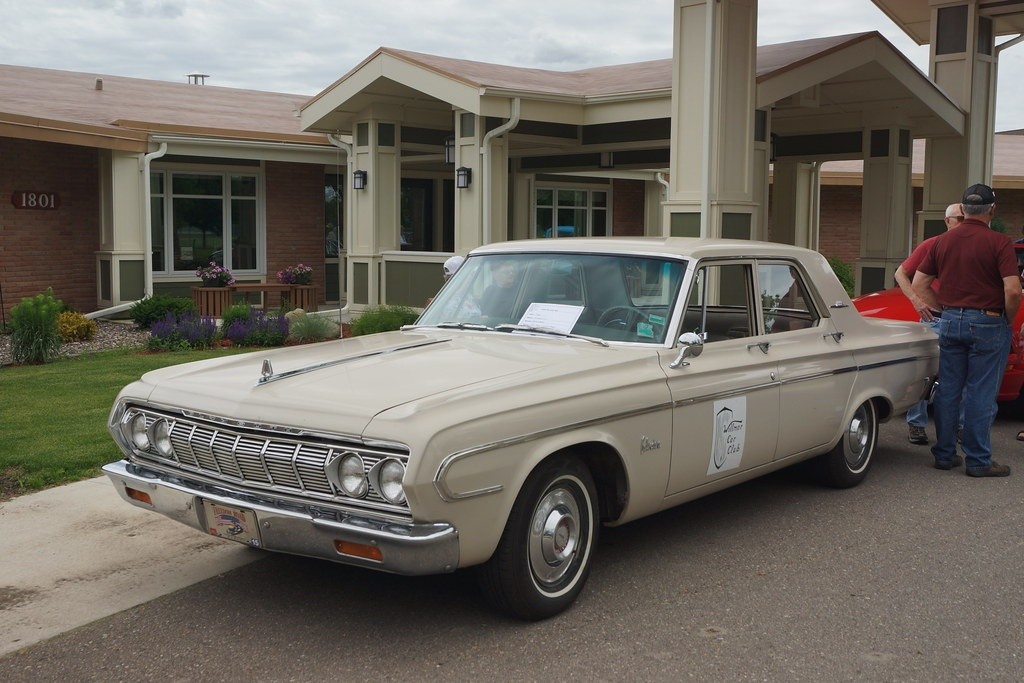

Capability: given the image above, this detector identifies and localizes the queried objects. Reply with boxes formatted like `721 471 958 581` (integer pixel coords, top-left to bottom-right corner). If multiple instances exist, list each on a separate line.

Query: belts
929 310 941 318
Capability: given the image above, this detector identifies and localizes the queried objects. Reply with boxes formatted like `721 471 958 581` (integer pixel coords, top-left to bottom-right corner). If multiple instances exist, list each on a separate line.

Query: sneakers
935 455 962 470
907 426 929 444
956 428 962 443
967 461 1010 477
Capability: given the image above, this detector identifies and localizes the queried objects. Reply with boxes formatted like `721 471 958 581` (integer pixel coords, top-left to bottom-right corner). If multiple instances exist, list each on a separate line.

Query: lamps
599 152 614 169
769 132 777 163
353 170 367 189
445 111 454 163
455 167 472 188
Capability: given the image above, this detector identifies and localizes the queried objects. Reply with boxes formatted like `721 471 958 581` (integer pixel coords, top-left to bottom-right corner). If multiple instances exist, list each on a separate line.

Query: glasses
948 216 964 222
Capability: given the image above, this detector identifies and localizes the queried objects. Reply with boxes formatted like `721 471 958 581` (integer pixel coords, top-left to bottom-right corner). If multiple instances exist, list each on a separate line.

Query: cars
851 241 1024 404
102 237 940 622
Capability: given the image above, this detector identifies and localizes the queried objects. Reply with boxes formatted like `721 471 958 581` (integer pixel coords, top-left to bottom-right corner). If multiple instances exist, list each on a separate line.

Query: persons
481 261 527 318
1016 431 1024 440
912 183 1023 477
436 255 482 321
894 204 964 446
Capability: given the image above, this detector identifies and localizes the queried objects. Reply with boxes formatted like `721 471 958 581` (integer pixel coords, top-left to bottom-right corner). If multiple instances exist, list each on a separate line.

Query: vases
202 279 226 287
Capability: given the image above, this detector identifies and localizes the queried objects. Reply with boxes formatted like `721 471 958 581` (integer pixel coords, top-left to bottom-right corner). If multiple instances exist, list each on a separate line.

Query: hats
962 183 996 205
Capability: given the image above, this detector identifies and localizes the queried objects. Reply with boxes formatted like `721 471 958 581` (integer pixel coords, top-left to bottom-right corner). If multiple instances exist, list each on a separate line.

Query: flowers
196 261 236 285
276 264 313 283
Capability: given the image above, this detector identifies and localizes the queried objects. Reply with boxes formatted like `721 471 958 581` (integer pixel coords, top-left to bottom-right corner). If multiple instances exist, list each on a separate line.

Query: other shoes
1016 430 1024 441
